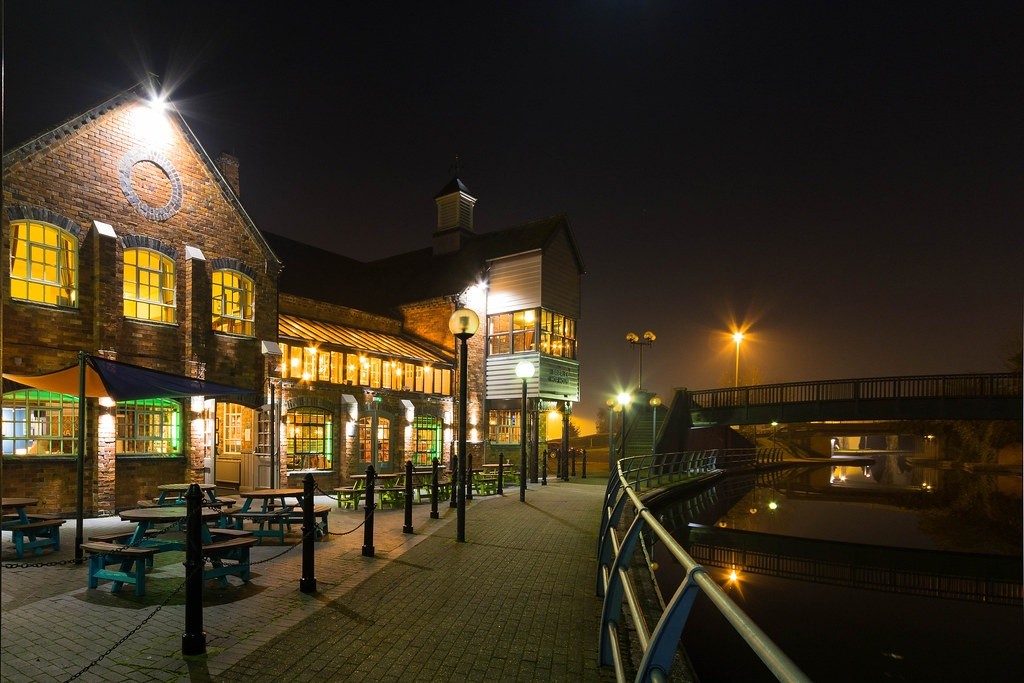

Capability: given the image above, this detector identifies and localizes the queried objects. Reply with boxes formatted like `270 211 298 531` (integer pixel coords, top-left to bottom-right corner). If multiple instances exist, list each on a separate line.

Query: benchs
2 470 520 599
446 469 484 479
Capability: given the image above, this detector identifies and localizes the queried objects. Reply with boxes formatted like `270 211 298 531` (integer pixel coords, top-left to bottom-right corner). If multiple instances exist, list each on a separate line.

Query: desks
481 463 514 474
395 471 432 484
2 498 42 556
415 466 446 478
111 509 228 592
240 488 303 514
349 475 397 489
157 483 217 505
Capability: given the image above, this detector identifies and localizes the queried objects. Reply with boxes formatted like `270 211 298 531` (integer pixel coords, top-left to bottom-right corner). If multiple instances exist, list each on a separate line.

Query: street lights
650 397 661 453
515 360 536 502
730 512 737 581
734 332 743 404
769 470 777 509
626 330 657 391
618 392 631 473
614 405 621 451
448 309 479 541
607 398 615 471
749 474 758 514
